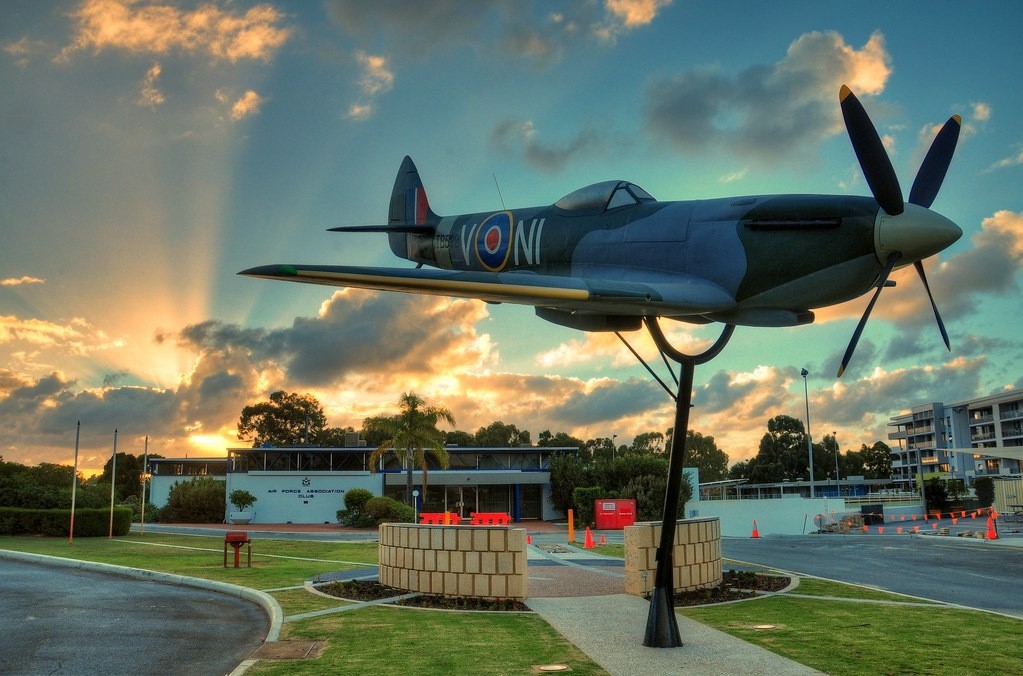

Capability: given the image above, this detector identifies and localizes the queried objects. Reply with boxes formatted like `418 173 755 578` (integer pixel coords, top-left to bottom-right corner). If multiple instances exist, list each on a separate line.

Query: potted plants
229 489 258 525
924 476 949 521
946 479 970 518
974 476 996 517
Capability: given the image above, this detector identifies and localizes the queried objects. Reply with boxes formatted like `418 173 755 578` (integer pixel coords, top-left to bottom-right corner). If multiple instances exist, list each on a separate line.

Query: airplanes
232 84 965 379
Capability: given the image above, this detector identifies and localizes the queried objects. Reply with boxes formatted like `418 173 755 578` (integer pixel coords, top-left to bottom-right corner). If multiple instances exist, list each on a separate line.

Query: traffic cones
527 534 532 543
583 526 605 549
987 518 996 539
749 519 763 538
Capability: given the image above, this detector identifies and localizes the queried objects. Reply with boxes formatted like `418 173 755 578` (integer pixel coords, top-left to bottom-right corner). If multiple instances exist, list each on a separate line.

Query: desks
1006 504 1023 522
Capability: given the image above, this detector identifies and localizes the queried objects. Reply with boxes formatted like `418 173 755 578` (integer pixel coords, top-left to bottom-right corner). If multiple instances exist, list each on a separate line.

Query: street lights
613 434 617 459
800 368 816 498
833 431 840 497
412 490 419 523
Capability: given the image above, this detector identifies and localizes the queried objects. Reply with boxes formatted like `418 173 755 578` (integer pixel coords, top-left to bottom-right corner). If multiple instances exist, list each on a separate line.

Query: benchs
999 510 1023 523
